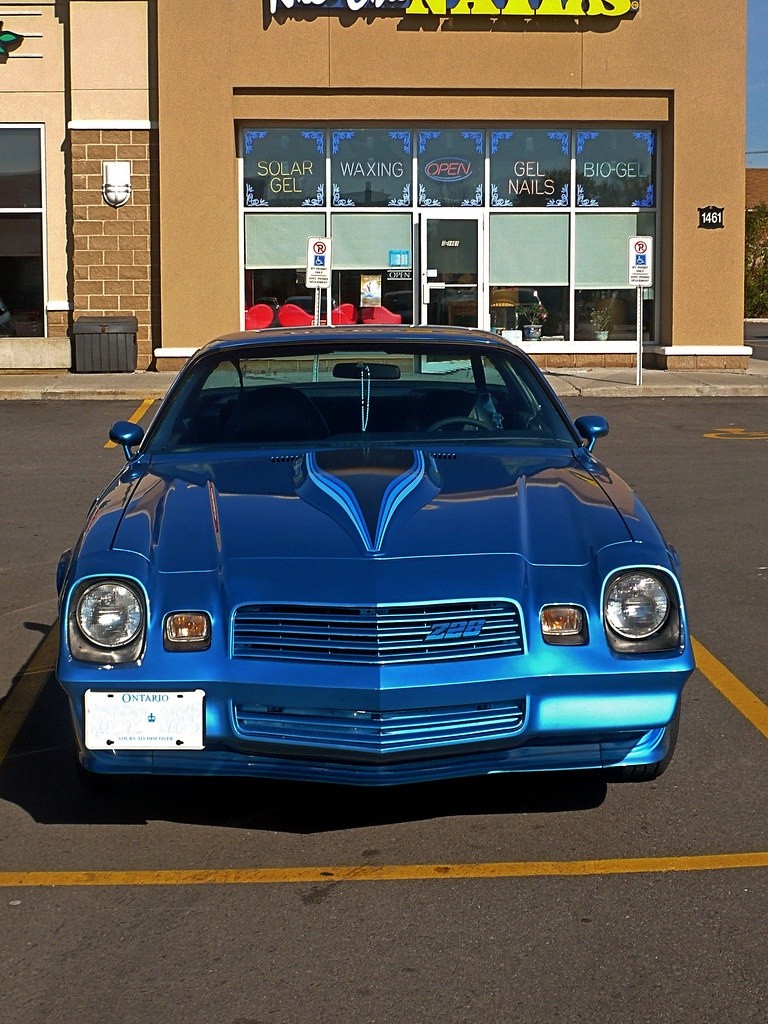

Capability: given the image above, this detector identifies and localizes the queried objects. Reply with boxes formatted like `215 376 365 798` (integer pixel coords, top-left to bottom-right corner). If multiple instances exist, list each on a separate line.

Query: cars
56 320 698 803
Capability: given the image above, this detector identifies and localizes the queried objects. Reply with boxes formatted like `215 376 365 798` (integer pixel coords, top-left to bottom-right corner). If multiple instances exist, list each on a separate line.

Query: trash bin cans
72 316 139 372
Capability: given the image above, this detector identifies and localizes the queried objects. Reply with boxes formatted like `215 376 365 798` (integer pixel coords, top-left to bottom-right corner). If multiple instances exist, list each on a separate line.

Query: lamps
100 161 132 207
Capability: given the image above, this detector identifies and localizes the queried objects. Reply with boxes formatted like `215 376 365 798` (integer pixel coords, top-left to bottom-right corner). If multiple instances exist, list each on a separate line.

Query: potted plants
513 303 548 341
590 307 614 341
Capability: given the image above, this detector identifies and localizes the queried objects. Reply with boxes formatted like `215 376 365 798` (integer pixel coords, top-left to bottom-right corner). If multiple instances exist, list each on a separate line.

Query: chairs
220 384 329 445
244 302 401 331
407 389 481 432
